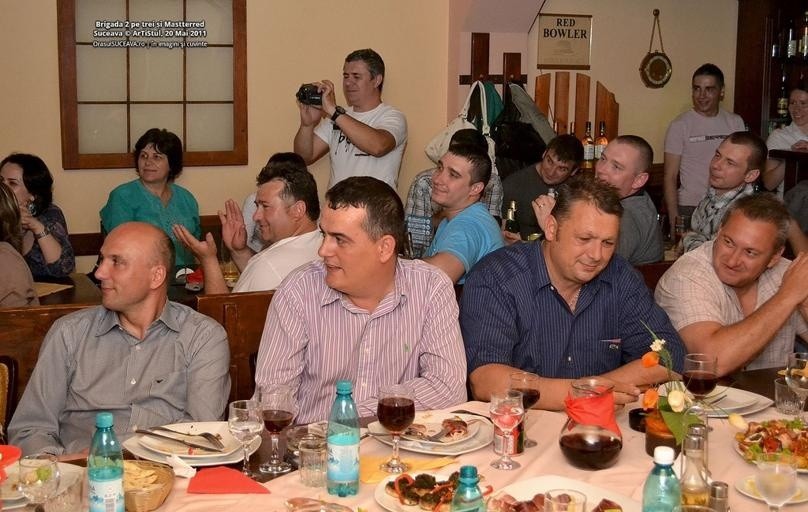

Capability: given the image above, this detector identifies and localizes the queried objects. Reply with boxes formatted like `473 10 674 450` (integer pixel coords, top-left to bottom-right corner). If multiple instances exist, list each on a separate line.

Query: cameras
296 84 322 105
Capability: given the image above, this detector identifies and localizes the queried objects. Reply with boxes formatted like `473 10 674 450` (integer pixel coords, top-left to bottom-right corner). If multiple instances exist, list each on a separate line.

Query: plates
368 410 494 457
494 475 642 511
653 382 774 420
120 420 262 469
733 478 808 504
372 472 492 511
1 458 84 511
733 431 808 473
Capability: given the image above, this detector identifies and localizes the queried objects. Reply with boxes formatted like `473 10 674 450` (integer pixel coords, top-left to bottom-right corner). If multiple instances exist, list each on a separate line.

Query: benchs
192 255 676 443
0 301 110 422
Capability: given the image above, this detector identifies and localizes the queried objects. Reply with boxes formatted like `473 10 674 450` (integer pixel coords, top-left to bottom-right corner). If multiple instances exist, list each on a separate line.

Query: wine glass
259 383 296 475
505 369 544 447
750 449 801 512
227 398 264 479
378 384 416 473
681 350 719 403
784 351 808 409
488 388 523 471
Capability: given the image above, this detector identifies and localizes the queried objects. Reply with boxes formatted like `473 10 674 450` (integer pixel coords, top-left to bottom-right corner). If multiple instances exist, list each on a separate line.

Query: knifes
135 428 222 453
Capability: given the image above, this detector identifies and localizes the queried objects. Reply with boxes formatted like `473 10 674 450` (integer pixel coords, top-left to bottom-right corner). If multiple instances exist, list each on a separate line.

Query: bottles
325 378 363 494
776 12 808 118
550 117 608 169
505 198 522 236
642 445 682 512
450 464 485 511
85 411 126 511
681 422 729 512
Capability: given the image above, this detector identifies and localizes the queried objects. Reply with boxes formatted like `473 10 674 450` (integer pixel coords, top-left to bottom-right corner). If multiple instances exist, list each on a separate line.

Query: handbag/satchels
495 84 538 161
422 80 497 178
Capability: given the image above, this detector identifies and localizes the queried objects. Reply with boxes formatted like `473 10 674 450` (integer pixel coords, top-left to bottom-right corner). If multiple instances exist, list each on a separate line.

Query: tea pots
558 375 623 471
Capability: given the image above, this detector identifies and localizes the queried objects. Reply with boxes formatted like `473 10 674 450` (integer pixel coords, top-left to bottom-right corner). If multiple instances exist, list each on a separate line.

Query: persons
760 84 808 199
1 125 807 461
662 63 748 243
294 48 409 203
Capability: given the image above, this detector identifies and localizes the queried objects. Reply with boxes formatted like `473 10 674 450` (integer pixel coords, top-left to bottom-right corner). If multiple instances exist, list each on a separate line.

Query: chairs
767 147 807 197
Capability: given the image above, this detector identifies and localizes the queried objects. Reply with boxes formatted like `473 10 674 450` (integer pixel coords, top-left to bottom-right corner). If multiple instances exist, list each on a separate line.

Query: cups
219 239 240 288
543 487 586 510
297 434 327 490
18 452 60 504
774 376 804 416
185 244 206 292
673 214 693 242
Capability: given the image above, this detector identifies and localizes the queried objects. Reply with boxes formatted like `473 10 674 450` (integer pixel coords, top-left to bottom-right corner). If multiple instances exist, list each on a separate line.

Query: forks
143 423 225 449
363 423 456 440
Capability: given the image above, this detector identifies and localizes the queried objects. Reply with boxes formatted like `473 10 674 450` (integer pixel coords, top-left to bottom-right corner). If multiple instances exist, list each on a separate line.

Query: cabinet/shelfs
733 0 807 142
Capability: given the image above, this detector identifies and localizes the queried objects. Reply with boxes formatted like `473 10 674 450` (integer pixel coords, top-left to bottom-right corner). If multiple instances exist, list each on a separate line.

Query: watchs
330 105 344 121
